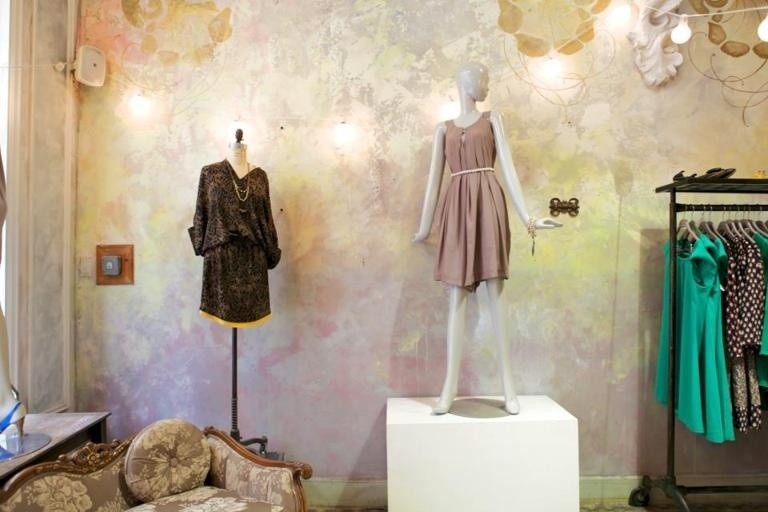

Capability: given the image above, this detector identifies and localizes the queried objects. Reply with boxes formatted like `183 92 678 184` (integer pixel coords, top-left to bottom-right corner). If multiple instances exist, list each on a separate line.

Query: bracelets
527 214 539 257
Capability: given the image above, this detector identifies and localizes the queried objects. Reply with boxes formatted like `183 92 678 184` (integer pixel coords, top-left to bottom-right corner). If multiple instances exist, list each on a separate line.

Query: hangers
676 203 768 244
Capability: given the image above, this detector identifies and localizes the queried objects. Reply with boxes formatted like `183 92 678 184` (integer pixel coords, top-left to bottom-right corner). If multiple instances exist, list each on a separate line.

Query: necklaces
225 159 251 203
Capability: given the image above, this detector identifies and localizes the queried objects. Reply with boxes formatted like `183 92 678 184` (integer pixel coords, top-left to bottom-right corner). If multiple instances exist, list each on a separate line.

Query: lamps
670 14 692 45
756 7 768 40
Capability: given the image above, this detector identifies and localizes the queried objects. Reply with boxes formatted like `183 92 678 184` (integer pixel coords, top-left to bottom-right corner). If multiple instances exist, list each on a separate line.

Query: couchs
0 426 313 512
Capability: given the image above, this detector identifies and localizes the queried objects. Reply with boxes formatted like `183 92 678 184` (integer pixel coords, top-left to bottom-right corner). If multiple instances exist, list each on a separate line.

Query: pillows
124 420 211 503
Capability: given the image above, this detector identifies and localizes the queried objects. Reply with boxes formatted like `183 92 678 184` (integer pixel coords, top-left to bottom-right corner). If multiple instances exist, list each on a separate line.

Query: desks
0 411 113 480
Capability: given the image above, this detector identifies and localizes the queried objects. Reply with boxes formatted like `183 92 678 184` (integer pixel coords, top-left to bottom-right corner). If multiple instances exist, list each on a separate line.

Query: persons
413 60 562 414
186 127 282 330
0 150 26 442
